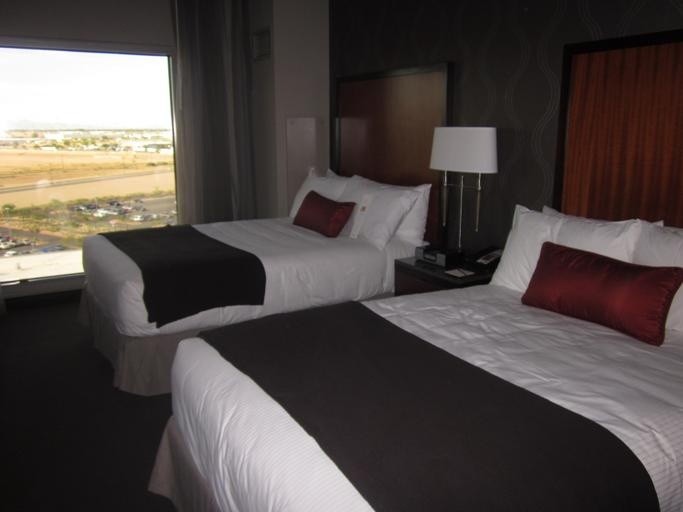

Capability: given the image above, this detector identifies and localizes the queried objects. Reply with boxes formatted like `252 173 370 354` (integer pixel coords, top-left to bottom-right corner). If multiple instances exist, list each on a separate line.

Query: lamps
426 123 499 265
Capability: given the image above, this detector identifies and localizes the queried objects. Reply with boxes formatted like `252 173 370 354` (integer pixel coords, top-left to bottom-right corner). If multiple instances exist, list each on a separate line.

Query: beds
80 167 434 398
145 203 683 512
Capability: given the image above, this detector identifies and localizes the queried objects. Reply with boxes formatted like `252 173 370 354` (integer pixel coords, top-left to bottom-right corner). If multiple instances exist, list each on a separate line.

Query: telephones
467 246 504 277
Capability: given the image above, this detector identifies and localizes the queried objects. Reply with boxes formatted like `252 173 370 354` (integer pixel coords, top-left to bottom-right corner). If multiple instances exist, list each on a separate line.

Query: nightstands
394 250 496 298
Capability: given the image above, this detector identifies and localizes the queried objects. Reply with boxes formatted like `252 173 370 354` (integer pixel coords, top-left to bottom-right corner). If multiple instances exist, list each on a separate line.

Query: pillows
496 204 683 347
289 168 432 251
